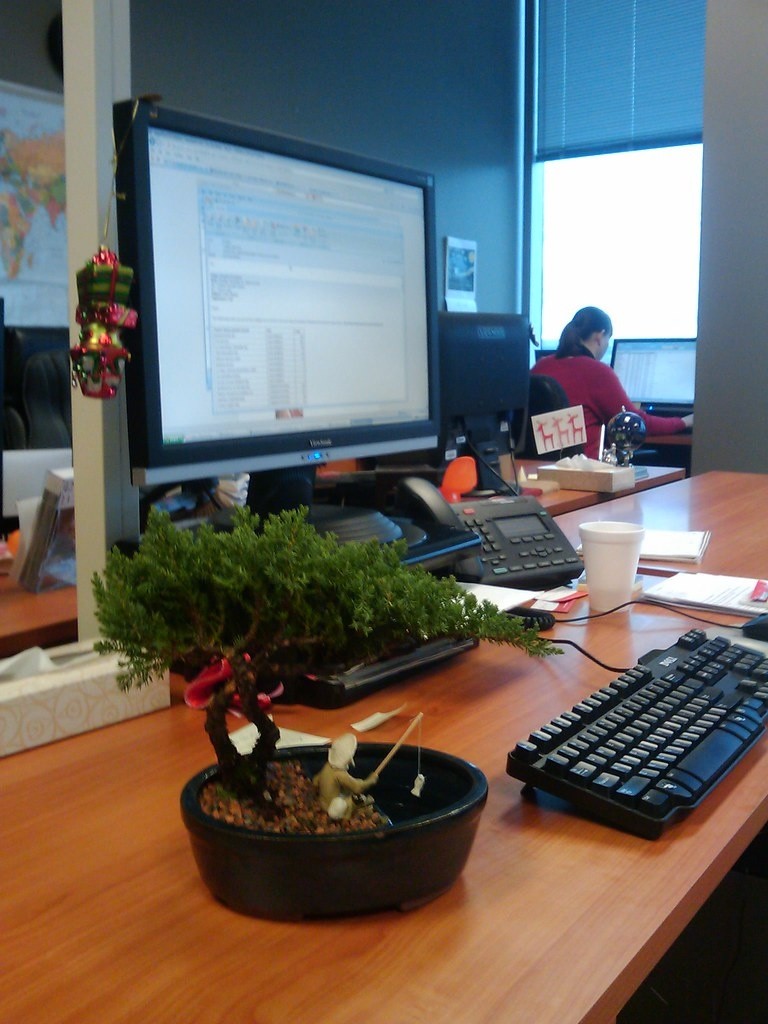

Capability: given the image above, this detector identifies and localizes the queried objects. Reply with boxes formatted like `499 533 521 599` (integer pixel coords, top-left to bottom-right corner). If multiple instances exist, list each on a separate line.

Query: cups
579 522 645 611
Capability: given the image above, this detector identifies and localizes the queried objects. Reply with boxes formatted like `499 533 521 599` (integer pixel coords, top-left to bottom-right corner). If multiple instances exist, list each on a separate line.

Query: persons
528 306 694 461
312 731 379 820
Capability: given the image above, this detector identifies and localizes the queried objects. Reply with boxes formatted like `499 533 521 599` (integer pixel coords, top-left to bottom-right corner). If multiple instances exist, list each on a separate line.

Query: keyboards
505 629 768 840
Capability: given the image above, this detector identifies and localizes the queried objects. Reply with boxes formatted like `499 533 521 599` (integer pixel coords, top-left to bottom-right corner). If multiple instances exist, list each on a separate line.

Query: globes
607 405 647 465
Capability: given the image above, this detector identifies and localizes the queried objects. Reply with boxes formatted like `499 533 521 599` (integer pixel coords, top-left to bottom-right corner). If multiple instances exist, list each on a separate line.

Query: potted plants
90 505 565 922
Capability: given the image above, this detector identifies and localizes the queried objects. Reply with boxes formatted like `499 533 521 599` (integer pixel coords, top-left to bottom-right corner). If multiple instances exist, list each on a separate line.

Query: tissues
0 637 171 757
537 453 635 492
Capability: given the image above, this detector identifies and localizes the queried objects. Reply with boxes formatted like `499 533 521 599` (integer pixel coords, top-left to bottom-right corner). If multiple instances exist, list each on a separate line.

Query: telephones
390 473 585 590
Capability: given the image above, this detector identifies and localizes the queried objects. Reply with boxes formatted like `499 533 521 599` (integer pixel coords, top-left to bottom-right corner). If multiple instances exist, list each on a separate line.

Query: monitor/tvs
110 97 441 546
609 338 697 419
436 310 531 498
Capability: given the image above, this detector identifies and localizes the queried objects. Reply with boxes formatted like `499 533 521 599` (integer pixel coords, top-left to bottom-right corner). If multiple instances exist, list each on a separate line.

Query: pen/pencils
598 421 607 462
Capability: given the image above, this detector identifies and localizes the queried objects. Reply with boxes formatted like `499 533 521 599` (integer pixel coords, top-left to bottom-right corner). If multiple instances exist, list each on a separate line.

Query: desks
1 460 767 1024
645 432 693 446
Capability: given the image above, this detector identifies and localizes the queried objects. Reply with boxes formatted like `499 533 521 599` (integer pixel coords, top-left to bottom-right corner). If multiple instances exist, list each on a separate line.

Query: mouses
742 613 768 642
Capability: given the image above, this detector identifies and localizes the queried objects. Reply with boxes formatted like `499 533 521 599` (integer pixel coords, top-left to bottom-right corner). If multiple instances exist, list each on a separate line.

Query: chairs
0 328 72 451
511 375 583 460
